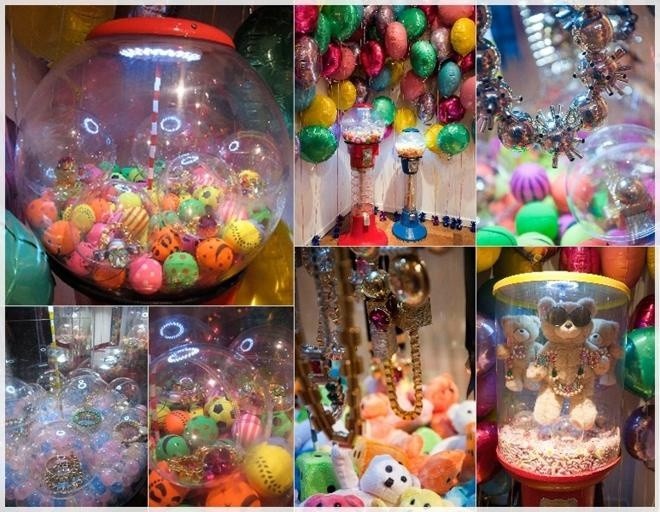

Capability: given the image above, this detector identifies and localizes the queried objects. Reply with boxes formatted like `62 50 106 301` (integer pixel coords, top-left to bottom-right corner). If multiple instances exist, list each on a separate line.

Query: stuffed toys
294 367 475 507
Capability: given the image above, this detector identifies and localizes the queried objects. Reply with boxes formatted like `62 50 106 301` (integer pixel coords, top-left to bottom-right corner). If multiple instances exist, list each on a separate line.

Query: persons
149 370 293 506
24 143 272 300
6 337 148 507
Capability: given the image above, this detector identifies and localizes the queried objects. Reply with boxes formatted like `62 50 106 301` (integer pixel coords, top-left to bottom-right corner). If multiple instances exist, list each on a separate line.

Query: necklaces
295 247 432 446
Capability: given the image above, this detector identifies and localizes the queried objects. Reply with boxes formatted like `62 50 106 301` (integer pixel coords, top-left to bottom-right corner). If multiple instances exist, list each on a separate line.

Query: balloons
476 122 655 246
294 5 475 163
477 247 655 484
